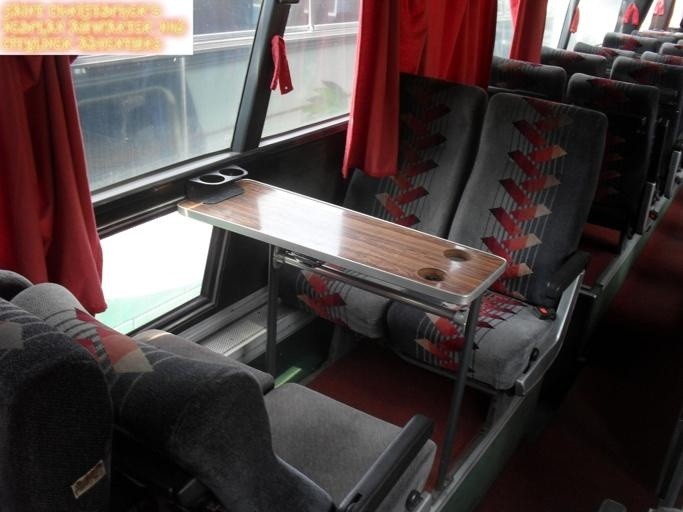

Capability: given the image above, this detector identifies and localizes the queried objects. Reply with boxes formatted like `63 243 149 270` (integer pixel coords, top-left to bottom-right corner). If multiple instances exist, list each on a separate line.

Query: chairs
0 26 683 512
